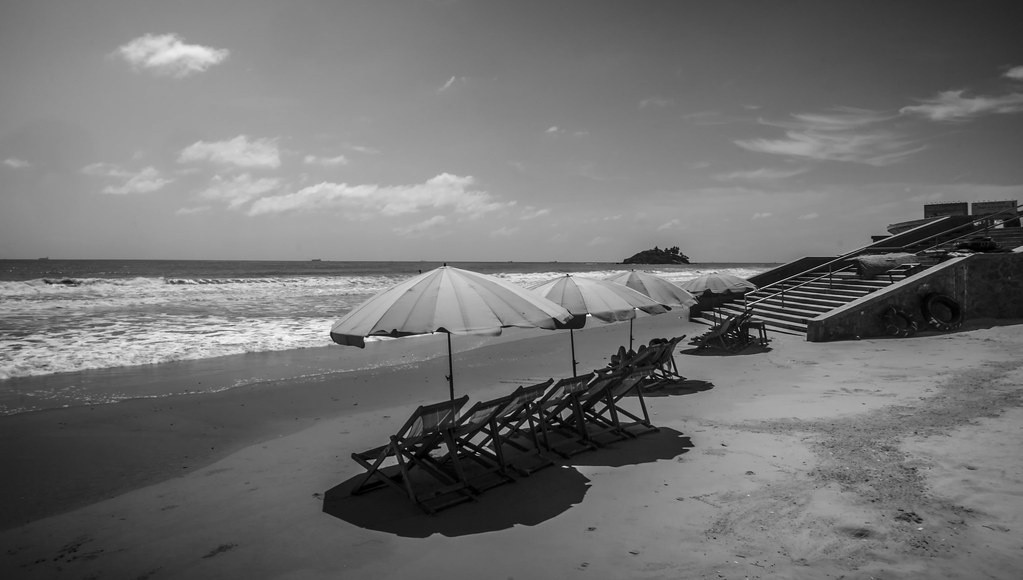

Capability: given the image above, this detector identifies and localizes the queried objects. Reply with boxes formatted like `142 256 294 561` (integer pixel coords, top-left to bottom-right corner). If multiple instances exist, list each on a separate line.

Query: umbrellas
601 269 698 358
330 263 573 478
526 274 674 377
680 273 758 329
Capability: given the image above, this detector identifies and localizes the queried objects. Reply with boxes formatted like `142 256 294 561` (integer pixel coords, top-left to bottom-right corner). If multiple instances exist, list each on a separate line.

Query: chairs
350 308 769 515
